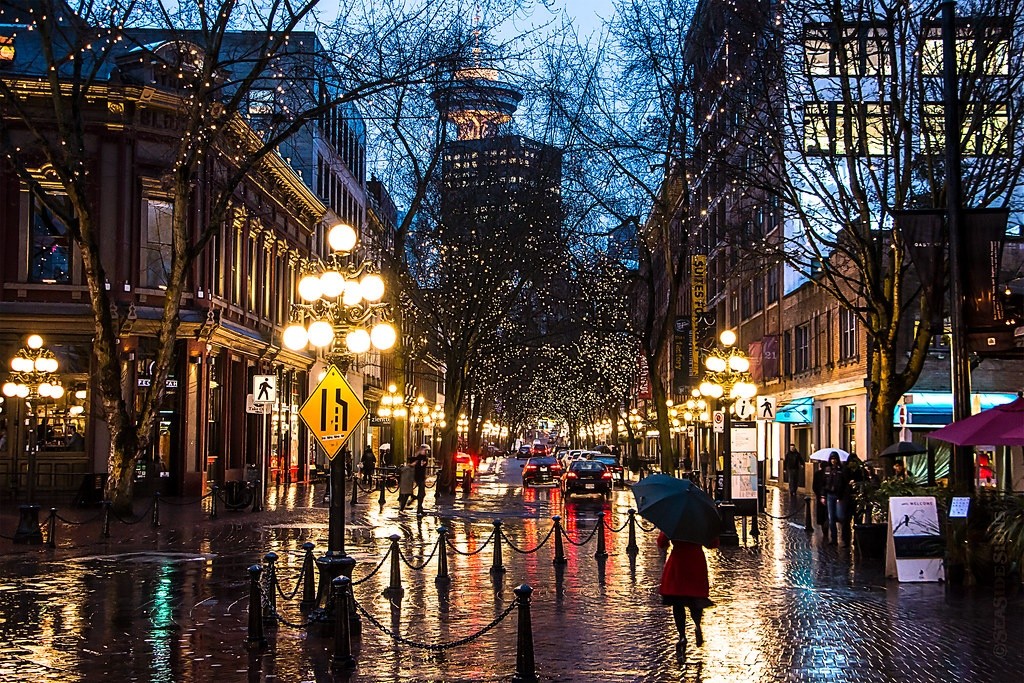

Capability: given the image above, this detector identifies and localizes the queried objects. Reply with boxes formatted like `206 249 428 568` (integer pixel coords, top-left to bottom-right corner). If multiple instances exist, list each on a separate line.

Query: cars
457 452 474 482
520 458 565 488
560 450 624 489
560 460 613 498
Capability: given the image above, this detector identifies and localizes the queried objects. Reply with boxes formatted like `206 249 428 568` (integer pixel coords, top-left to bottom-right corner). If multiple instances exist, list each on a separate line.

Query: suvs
550 445 568 456
517 446 532 459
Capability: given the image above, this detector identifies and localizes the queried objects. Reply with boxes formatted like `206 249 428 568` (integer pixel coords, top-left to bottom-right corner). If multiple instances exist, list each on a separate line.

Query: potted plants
852 477 943 560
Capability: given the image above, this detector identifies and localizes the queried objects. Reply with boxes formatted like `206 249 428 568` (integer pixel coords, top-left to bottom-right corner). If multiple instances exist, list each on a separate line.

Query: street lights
2 334 64 543
456 413 469 452
284 225 396 637
410 395 430 447
430 405 447 458
594 408 643 467
481 420 508 446
684 388 707 483
378 385 406 468
699 332 757 546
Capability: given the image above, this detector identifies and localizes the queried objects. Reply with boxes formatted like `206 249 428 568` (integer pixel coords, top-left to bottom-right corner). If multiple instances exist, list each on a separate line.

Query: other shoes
404 506 413 510
828 542 838 546
842 541 850 548
398 511 408 516
695 626 702 647
676 637 687 652
417 509 427 516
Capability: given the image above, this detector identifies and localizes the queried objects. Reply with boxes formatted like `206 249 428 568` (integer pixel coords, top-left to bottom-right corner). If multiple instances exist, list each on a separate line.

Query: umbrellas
811 443 850 461
631 476 723 545
921 390 1024 445
879 441 930 461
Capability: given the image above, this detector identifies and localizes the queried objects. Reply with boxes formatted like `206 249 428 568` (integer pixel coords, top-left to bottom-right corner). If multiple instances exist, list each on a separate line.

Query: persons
38 417 82 450
657 531 721 652
344 444 432 517
698 444 914 549
0 428 7 451
977 451 992 488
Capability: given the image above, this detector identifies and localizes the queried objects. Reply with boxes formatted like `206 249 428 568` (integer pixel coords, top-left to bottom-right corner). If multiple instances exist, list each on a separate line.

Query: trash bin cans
682 473 699 489
225 480 247 509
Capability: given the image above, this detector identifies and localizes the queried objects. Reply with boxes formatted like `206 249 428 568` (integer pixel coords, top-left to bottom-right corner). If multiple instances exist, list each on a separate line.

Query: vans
532 444 546 457
